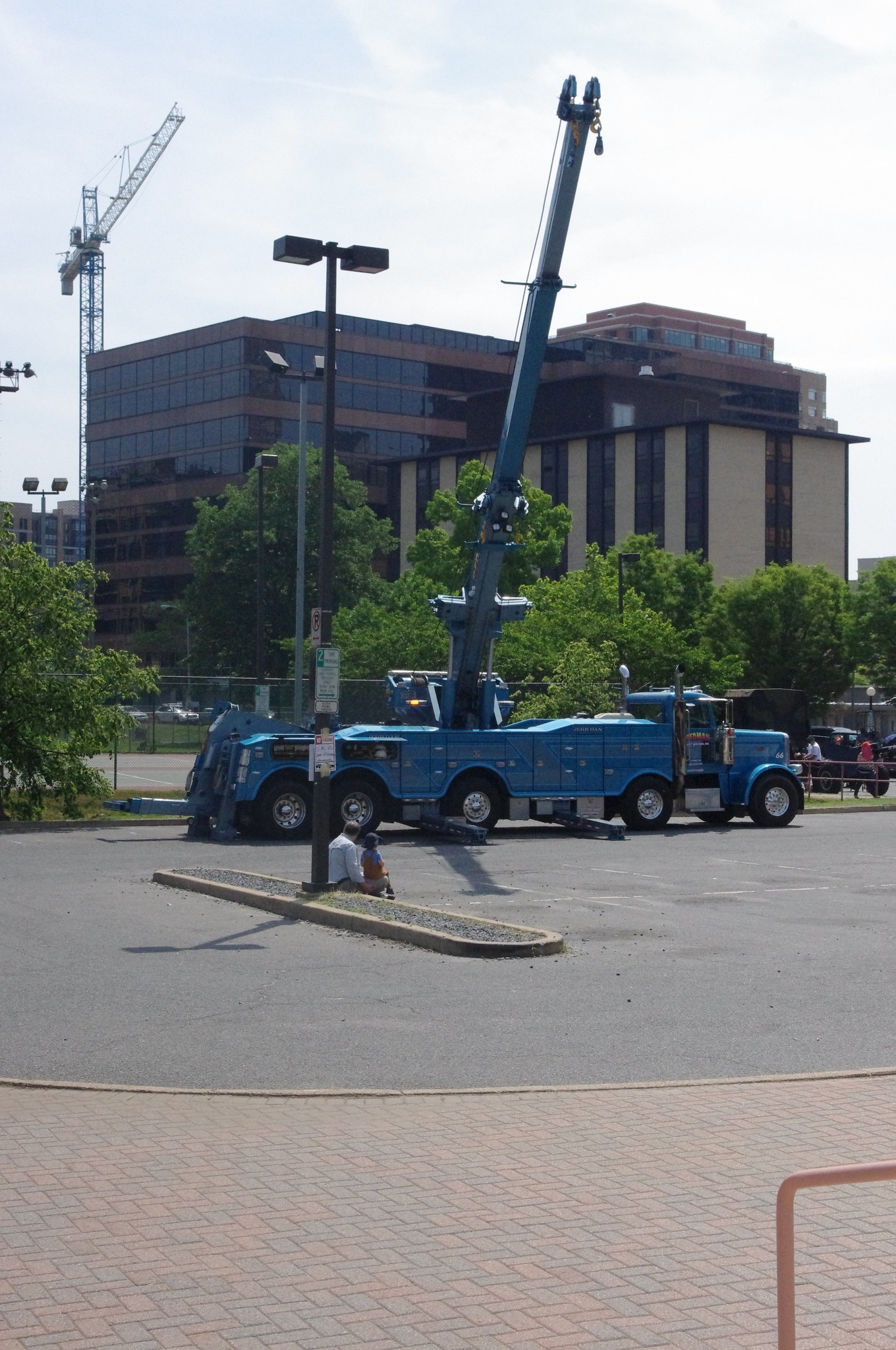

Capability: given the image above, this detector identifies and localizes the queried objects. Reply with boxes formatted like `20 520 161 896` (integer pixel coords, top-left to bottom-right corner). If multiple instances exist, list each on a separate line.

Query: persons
801 735 822 792
685 705 711 729
193 763 223 842
328 820 389 896
360 832 394 895
835 737 841 745
853 742 880 799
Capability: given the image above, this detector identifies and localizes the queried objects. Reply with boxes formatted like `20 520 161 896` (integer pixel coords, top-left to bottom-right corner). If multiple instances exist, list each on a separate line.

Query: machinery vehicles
185 74 805 841
793 725 890 797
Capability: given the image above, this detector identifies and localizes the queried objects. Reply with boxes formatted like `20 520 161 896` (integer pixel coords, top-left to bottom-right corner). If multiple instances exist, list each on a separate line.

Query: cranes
52 101 185 592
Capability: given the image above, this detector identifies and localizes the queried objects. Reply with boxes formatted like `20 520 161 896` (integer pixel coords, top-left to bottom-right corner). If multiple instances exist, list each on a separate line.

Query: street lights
618 551 641 667
160 604 191 696
78 478 108 648
21 477 69 562
275 235 388 704
866 686 876 710
253 454 279 685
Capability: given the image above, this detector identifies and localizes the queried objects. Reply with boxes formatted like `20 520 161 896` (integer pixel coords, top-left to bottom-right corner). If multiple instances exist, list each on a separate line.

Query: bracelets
703 721 705 725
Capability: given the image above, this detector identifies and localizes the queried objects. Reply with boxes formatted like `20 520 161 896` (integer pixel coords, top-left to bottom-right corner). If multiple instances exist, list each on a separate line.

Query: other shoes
369 892 386 898
386 888 394 894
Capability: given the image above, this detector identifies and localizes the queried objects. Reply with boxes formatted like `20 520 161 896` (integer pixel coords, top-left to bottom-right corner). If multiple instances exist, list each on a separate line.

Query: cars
153 704 199 724
119 705 148 724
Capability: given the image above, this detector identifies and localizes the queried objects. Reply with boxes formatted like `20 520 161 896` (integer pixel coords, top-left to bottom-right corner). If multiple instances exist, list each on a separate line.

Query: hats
806 736 813 742
862 742 872 746
361 833 383 849
